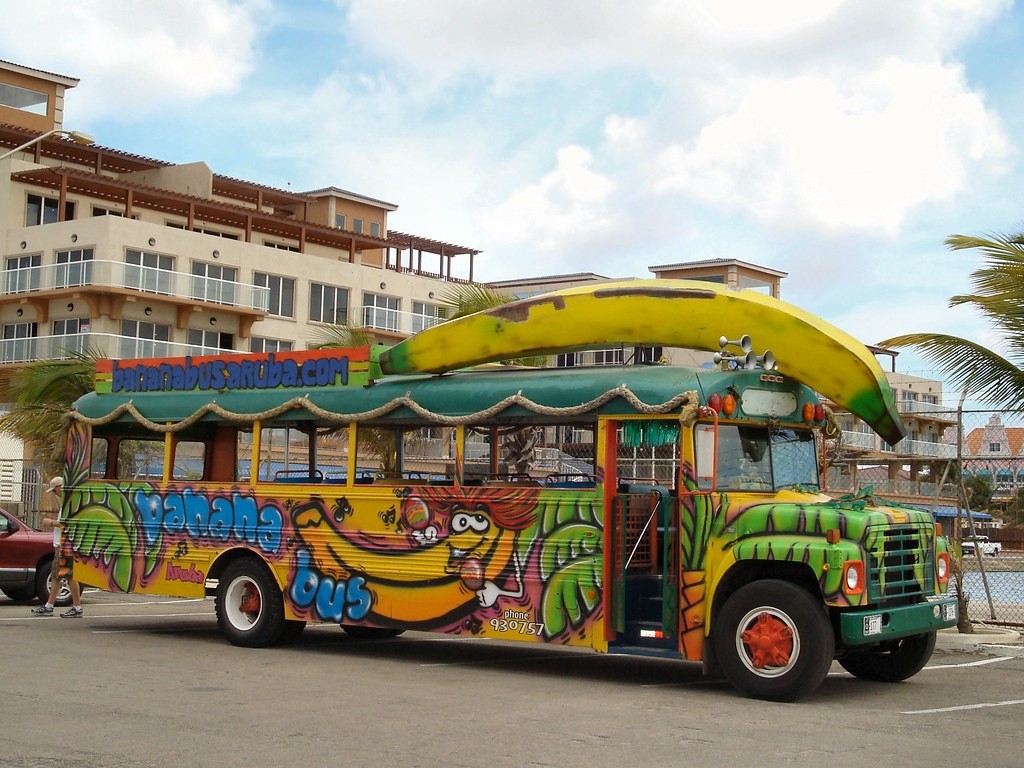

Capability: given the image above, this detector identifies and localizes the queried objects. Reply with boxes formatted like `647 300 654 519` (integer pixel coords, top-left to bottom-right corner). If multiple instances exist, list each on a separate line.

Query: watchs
49 520 54 527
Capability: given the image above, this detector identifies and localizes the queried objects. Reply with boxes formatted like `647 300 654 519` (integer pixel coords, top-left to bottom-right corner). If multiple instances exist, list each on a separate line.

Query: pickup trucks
962 535 1002 558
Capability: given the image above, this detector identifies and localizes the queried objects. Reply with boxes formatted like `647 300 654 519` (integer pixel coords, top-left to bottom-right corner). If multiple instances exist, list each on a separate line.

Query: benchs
274 469 672 495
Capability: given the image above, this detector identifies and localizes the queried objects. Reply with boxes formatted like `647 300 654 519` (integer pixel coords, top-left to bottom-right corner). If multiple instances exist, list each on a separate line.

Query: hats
46 476 63 492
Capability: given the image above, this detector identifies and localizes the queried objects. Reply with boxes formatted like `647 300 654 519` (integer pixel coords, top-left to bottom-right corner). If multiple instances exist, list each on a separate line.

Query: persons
31 476 83 619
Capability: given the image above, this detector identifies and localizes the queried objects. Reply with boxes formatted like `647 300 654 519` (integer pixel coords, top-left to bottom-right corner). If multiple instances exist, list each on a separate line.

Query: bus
58 278 964 701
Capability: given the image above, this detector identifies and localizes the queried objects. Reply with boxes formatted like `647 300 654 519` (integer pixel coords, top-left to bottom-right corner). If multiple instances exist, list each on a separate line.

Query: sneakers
60 607 83 618
31 602 54 616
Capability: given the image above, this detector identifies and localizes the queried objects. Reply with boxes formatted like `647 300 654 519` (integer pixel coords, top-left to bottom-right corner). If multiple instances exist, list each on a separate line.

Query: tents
901 503 993 545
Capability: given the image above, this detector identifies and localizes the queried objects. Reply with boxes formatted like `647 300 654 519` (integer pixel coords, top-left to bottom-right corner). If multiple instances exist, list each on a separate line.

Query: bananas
377 278 908 446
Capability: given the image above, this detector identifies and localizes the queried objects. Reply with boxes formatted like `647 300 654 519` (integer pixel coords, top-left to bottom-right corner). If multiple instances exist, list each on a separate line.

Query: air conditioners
904 420 919 431
924 423 939 433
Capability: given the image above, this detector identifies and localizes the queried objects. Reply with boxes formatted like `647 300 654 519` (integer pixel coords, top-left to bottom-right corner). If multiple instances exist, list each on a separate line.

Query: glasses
53 488 58 493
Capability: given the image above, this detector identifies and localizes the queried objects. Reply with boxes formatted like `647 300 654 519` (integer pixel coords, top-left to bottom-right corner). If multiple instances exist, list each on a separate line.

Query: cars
0 507 84 606
